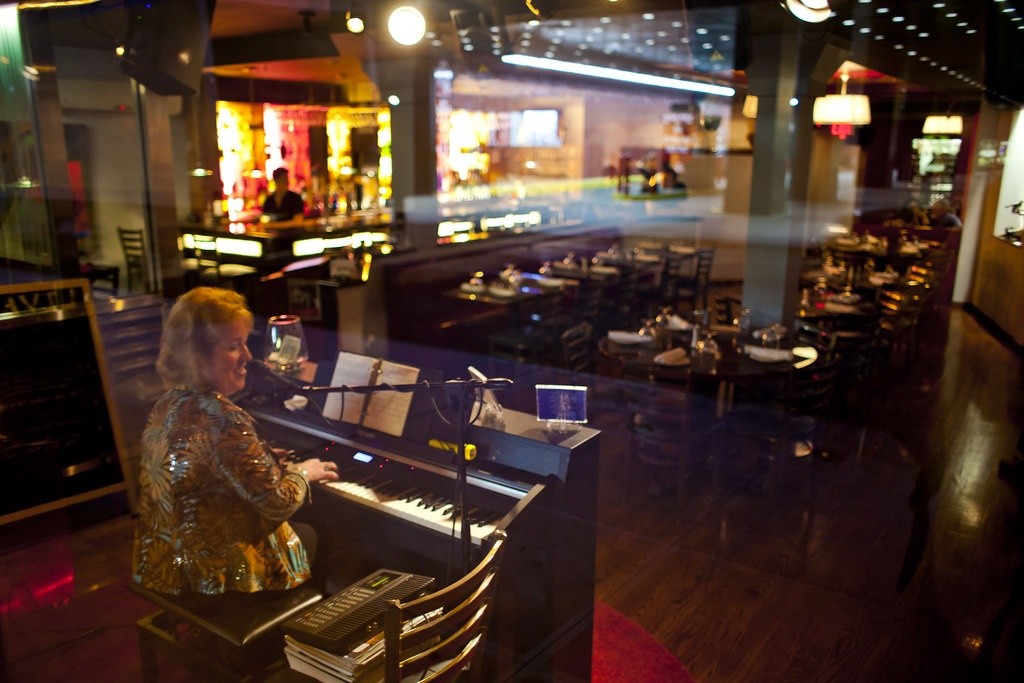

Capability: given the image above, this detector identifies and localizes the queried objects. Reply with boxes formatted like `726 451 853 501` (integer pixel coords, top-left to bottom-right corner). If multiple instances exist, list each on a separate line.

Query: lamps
813 73 872 140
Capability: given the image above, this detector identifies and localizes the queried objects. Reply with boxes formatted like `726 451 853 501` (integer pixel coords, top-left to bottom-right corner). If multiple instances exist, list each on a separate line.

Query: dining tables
531 252 618 285
633 241 701 261
599 305 826 381
799 264 941 293
591 246 661 273
825 236 939 266
441 279 581 361
727 273 869 320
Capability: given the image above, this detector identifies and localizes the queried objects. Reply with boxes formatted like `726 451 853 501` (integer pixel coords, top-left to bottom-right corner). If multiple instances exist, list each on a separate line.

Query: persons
634 146 687 196
928 198 963 229
128 286 342 613
259 168 305 230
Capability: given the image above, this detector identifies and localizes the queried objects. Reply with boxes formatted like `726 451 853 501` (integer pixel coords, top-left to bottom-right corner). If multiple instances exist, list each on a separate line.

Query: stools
79 264 118 297
128 577 323 683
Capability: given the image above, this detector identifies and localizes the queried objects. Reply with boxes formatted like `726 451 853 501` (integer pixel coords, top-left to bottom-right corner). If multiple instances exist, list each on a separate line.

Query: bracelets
296 465 308 476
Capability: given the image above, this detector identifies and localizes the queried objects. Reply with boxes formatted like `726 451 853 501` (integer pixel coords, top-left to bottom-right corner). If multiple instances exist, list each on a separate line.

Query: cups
261 315 308 373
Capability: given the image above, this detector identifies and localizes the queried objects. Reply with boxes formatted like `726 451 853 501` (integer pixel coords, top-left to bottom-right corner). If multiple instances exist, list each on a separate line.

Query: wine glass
761 322 787 350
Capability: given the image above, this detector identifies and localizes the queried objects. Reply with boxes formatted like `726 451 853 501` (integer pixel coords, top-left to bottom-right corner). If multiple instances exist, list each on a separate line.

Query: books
283 607 444 683
321 350 420 437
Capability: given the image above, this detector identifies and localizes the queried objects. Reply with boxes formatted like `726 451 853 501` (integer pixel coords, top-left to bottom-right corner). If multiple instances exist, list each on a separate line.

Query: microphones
249 360 309 397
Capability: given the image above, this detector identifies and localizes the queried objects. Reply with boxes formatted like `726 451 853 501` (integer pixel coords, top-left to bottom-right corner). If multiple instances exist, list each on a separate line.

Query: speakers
114 0 217 95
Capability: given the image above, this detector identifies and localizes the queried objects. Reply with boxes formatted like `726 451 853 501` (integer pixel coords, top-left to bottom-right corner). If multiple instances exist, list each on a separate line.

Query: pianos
232 351 601 682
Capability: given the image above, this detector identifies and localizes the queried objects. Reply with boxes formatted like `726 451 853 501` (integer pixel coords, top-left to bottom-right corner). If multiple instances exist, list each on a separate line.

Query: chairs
267 529 507 683
116 227 155 293
489 239 950 500
